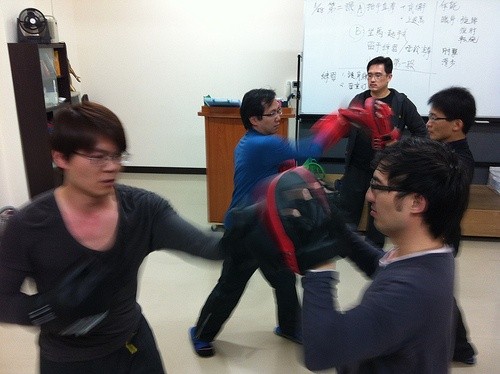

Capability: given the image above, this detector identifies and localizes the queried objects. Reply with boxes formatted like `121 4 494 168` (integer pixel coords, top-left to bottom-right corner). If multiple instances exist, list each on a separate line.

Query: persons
375 84 479 367
0 102 269 374
291 135 468 374
188 88 348 357
334 58 431 248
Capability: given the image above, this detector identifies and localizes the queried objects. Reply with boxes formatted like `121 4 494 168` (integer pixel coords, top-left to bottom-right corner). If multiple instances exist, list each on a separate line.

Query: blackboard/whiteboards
298 0 500 119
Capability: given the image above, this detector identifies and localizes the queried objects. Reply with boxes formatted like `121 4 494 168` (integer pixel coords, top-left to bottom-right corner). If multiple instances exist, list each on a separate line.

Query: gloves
312 113 351 149
28 276 111 339
219 203 261 257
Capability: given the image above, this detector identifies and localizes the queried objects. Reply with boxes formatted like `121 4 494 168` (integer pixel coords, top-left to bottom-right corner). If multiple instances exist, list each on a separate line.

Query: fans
16 8 51 45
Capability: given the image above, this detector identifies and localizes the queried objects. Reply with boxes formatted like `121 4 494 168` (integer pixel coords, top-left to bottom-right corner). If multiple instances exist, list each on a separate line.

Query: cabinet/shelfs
7 42 80 200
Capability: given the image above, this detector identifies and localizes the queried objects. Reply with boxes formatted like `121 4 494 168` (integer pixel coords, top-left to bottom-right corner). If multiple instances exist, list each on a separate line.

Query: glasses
365 73 388 80
251 101 283 117
70 150 131 167
370 178 420 194
428 114 458 123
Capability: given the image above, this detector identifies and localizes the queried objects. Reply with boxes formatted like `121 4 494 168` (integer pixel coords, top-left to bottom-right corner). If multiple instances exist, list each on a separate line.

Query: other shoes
451 342 476 365
276 326 304 345
190 327 215 358
